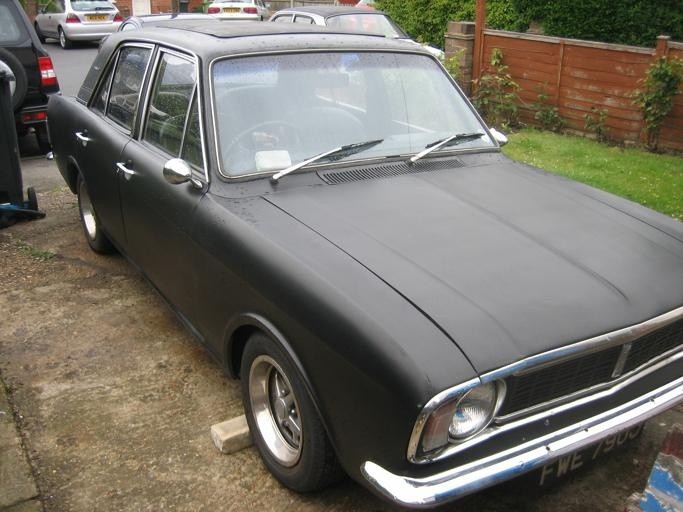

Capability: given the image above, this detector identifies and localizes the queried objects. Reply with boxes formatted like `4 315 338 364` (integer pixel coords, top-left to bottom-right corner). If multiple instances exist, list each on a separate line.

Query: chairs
108 84 368 169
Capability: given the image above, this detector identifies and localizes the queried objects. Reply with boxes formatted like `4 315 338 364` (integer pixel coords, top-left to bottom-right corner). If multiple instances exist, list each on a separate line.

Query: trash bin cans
0 70 38 227
